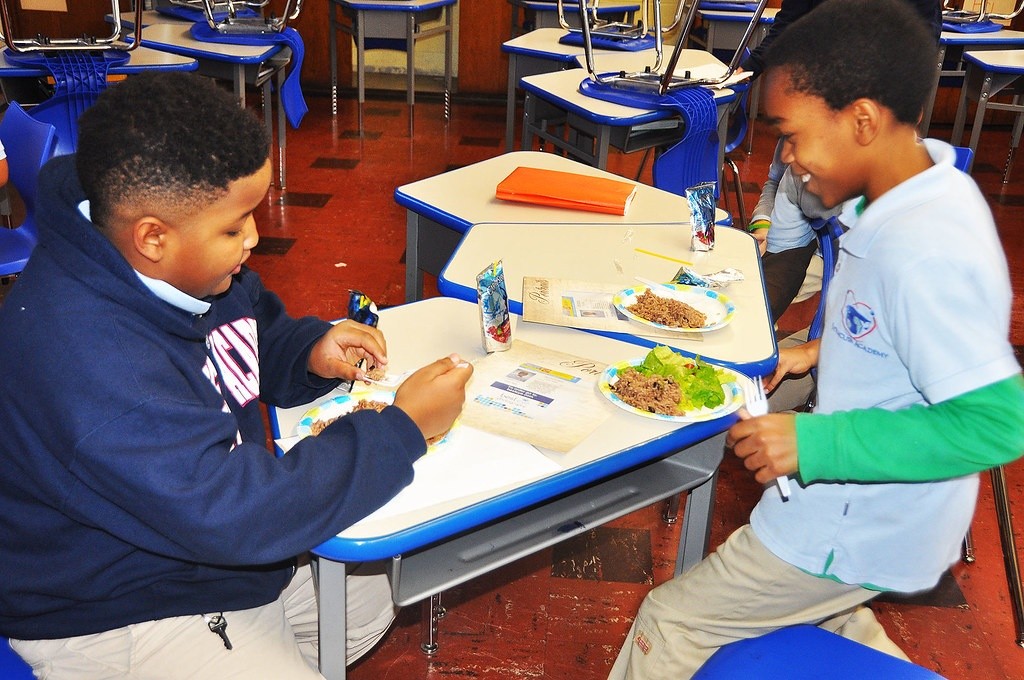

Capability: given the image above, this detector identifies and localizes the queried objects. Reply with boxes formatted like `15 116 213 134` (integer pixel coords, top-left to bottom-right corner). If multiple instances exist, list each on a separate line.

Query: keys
208 616 233 650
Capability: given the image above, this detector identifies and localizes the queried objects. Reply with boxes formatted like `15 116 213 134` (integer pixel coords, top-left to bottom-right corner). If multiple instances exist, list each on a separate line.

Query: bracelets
749 224 770 230
754 220 771 225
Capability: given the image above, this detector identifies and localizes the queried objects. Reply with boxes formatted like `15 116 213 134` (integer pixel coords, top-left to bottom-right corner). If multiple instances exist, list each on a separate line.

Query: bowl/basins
296 390 445 447
612 283 734 333
597 358 745 422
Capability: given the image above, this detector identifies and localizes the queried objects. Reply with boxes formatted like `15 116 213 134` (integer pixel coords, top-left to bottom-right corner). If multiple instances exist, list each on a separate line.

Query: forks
745 375 790 497
361 362 470 388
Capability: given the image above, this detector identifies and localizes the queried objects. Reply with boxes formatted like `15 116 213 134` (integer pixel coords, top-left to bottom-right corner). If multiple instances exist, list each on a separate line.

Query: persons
607 0 1024 680
749 135 847 413
0 70 474 680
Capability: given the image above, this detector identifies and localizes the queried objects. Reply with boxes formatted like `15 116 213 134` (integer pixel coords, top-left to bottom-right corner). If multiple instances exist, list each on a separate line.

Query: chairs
0 0 1024 381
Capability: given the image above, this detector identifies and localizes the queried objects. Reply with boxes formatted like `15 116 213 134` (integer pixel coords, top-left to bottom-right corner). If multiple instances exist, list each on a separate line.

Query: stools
693 624 948 680
940 0 1024 34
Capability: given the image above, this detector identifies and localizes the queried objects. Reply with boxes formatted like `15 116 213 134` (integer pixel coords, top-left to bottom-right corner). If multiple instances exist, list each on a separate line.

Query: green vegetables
616 344 733 411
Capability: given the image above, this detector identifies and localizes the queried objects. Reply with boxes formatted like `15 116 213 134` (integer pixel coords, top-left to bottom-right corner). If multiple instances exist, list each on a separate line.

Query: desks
0 0 780 680
950 50 1024 174
917 30 1024 146
